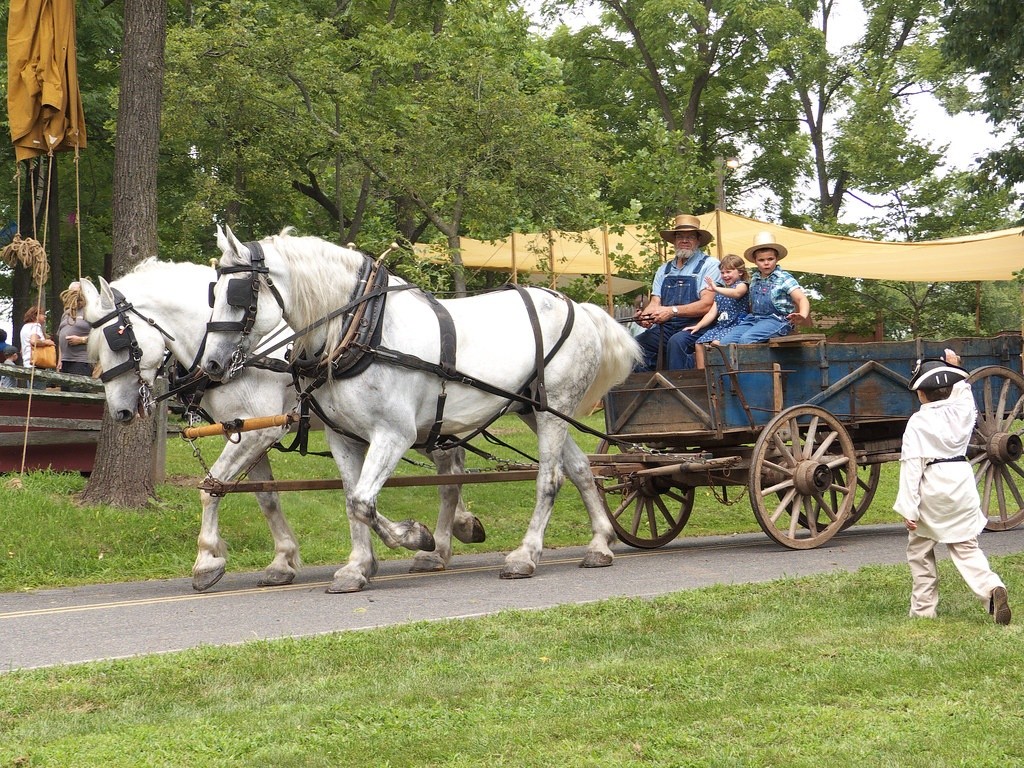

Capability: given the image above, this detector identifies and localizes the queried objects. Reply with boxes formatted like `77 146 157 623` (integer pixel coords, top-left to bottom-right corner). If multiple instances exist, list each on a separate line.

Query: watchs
672 306 678 317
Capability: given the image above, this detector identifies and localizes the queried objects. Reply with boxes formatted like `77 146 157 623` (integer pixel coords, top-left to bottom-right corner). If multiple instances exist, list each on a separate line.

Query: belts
925 455 968 467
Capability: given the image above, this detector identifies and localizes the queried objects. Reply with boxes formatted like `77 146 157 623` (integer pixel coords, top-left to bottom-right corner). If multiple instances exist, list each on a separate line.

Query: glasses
675 235 699 241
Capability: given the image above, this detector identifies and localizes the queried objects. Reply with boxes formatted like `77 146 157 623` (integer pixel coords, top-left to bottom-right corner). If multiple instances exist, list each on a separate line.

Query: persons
632 215 810 371
892 348 1011 626
57 281 92 392
0 329 18 387
20 305 56 390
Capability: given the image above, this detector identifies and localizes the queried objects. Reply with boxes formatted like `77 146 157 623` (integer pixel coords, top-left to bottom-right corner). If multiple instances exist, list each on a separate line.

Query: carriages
60 224 1024 594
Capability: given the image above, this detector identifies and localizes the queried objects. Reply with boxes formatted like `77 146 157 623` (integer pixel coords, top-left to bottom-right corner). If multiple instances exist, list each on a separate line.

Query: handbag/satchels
29 326 57 369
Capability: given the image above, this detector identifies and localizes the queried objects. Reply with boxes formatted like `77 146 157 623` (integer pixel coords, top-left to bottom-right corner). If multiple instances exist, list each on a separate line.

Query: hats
908 357 970 391
660 215 714 248
744 233 787 263
3 346 21 355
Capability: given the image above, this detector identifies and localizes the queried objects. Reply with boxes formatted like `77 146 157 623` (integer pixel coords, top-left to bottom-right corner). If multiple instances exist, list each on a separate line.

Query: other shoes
993 586 1011 625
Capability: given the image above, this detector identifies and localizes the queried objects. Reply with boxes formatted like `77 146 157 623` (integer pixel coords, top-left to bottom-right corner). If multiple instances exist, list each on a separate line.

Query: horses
74 222 646 595
